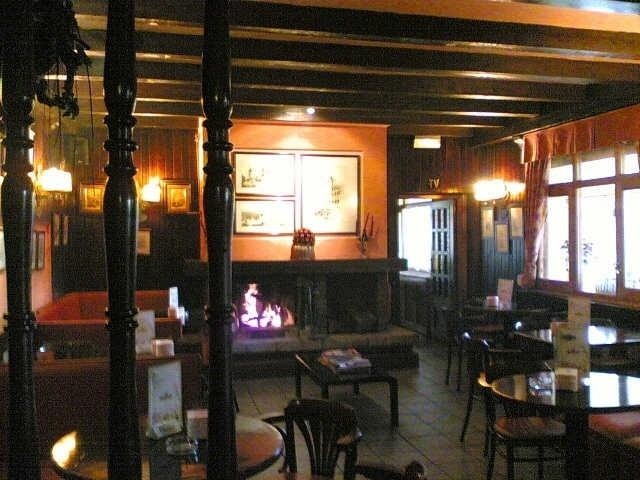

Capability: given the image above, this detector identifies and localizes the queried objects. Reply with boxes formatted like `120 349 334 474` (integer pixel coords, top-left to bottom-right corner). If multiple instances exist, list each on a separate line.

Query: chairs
437 294 640 480
266 398 362 480
355 459 426 479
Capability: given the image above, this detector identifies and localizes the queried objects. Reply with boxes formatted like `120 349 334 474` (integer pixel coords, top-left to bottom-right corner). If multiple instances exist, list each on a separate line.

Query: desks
51 408 284 480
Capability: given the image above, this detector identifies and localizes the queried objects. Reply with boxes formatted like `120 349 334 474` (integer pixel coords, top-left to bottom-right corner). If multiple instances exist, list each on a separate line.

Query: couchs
1 330 199 462
37 289 179 339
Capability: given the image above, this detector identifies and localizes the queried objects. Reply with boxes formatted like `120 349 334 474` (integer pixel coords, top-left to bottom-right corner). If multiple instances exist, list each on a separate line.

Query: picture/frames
299 149 361 236
80 185 104 214
32 231 36 269
230 151 296 197
37 230 45 271
161 179 192 217
235 198 297 236
